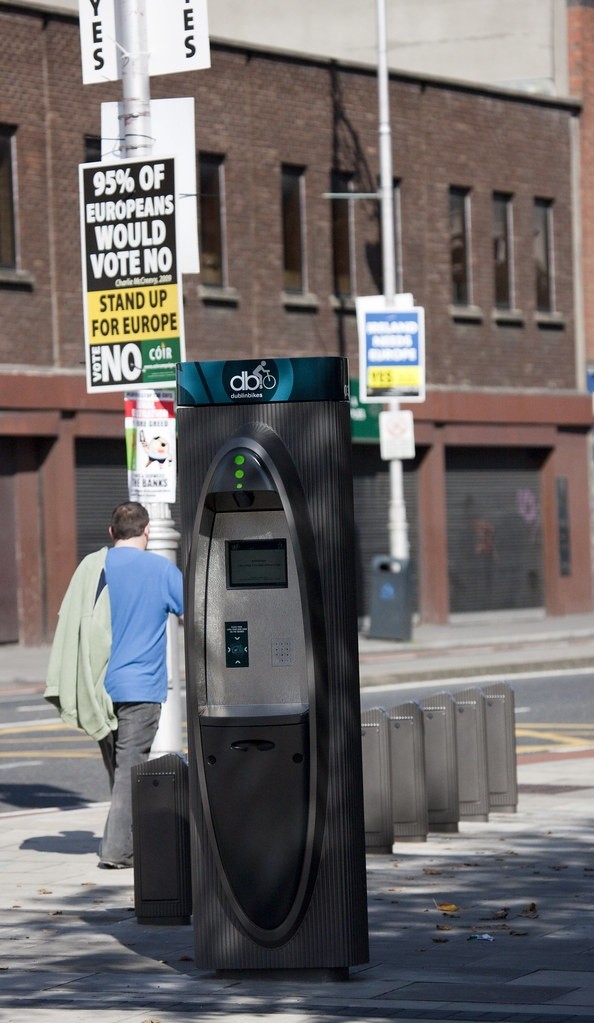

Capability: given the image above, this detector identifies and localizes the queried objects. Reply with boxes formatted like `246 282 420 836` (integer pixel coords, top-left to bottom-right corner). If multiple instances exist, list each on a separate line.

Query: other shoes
98 860 130 869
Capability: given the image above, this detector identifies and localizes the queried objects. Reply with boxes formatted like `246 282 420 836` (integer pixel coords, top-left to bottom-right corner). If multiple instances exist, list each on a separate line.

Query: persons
79 501 185 869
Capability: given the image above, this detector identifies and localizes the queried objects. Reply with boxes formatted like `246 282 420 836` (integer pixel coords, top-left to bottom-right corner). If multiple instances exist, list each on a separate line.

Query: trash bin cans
366 553 413 643
